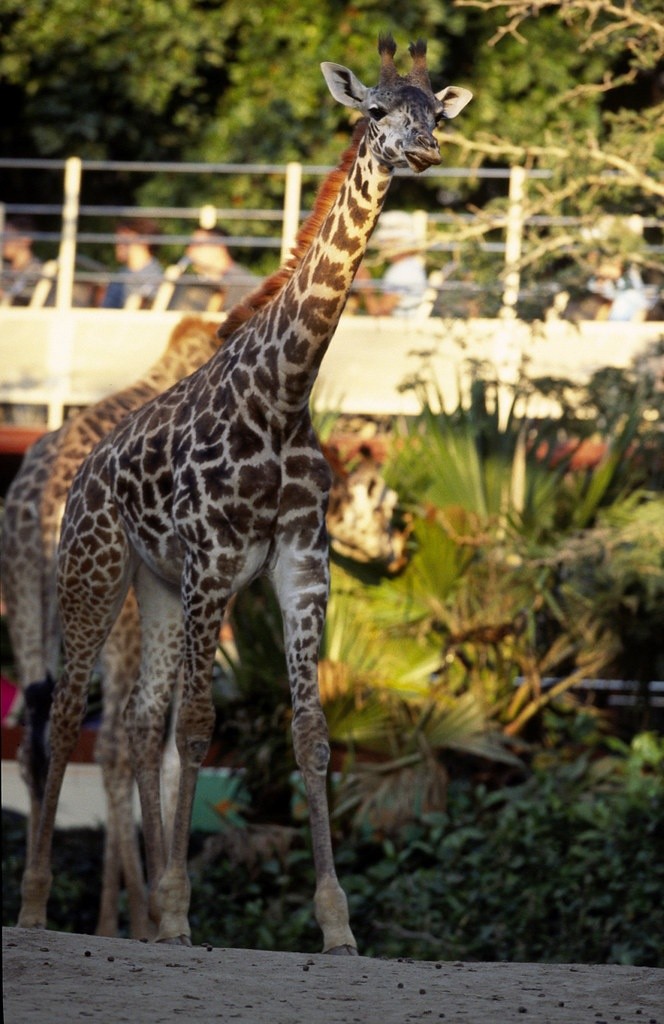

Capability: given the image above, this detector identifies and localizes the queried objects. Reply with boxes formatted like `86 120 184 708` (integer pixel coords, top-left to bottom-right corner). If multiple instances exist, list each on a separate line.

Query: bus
0 154 664 894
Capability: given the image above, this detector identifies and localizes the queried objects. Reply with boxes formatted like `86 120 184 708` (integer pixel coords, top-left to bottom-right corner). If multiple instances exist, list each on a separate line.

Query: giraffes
18 30 474 956
0 317 397 941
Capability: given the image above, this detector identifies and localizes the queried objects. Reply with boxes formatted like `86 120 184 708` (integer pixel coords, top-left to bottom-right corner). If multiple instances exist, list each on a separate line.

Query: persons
2 222 251 313
369 210 664 319
